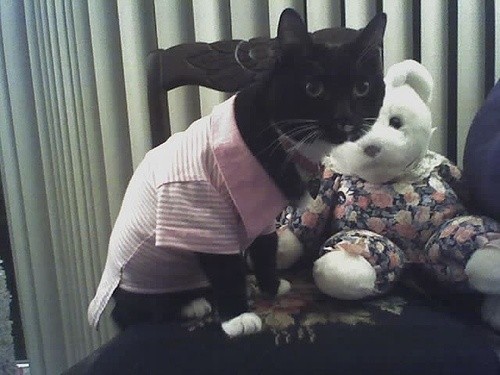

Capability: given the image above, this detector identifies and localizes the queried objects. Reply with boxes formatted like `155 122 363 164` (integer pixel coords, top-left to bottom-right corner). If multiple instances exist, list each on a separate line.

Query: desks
57 323 500 375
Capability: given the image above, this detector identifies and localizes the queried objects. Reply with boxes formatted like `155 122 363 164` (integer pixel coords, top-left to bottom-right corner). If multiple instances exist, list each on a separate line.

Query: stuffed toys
276 57 500 302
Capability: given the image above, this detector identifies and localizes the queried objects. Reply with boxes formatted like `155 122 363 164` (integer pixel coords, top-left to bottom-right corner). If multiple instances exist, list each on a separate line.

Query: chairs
143 26 364 147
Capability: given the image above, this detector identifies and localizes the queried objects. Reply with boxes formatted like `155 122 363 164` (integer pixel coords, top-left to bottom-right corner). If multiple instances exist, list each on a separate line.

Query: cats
110 8 387 337
